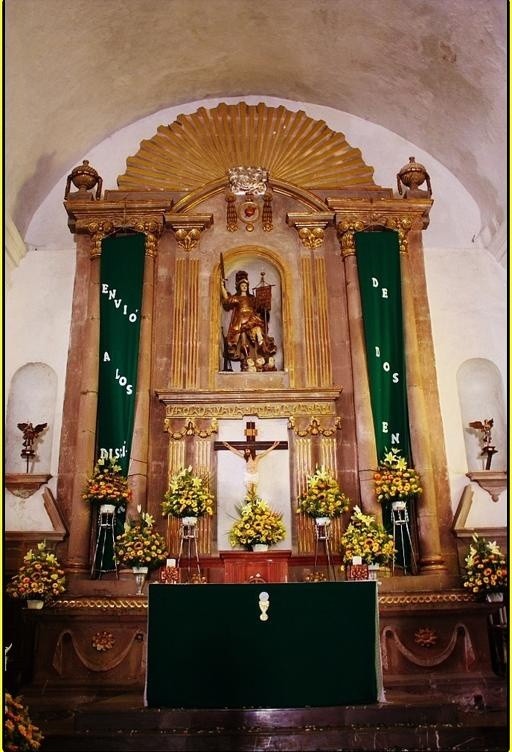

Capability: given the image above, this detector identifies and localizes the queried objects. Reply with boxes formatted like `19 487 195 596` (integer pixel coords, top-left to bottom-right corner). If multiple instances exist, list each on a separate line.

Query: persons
23 422 38 447
219 276 279 357
482 418 492 447
221 439 281 496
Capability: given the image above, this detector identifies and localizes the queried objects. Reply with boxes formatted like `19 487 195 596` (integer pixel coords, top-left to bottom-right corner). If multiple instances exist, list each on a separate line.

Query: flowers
81 449 132 510
227 489 285 547
373 445 423 503
4 537 68 600
341 506 397 563
294 462 350 521
460 530 508 592
113 504 168 565
161 466 215 520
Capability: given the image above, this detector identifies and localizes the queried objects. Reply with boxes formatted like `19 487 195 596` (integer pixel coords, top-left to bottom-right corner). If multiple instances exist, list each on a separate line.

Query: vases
485 590 504 603
27 598 43 609
